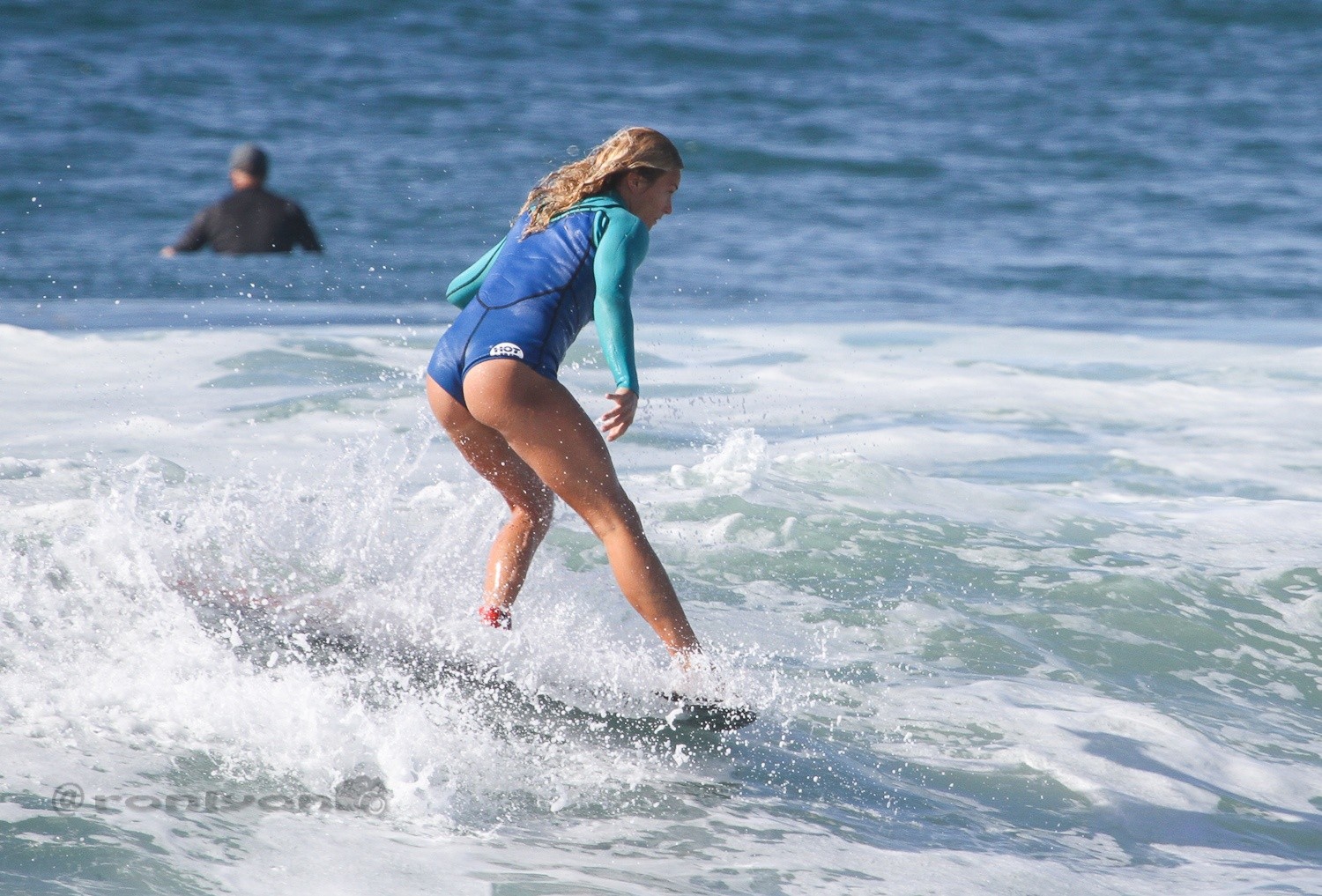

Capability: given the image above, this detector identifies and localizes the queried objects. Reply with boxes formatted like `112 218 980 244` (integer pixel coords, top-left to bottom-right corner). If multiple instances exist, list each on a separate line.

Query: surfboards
474 640 758 732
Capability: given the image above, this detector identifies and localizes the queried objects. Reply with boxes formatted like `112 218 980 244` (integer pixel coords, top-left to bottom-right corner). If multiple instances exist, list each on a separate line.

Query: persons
160 142 320 256
425 124 737 701
